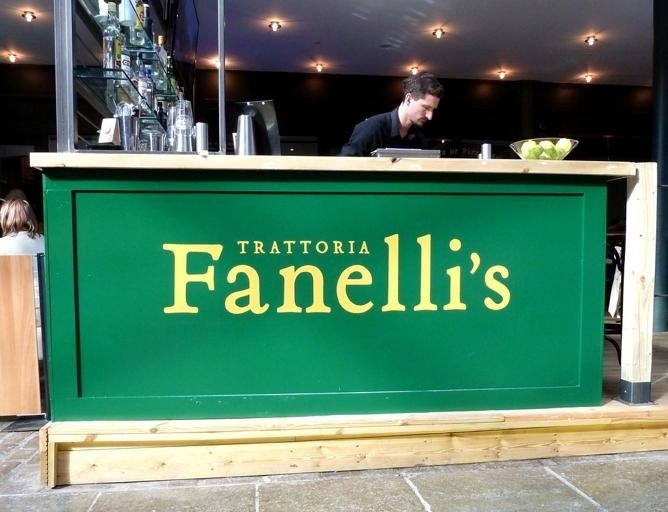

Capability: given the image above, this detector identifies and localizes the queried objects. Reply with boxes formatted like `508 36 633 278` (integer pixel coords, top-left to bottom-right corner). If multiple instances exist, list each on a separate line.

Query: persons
0 197 44 254
339 72 444 156
4 189 27 200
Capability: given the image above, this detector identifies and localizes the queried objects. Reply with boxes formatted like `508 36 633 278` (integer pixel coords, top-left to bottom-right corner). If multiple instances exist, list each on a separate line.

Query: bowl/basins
509 138 579 160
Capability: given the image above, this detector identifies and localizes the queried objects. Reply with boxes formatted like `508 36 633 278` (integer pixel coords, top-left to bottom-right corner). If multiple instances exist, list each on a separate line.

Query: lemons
521 138 572 158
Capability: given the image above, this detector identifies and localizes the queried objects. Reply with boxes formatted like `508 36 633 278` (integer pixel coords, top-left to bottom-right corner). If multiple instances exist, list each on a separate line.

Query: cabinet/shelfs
53 0 196 152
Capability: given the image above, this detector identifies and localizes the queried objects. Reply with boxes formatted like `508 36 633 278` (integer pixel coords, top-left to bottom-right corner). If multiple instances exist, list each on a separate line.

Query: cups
232 132 237 152
476 143 492 160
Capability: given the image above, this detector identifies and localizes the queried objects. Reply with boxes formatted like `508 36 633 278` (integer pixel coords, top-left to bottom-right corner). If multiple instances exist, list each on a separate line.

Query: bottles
235 114 257 156
97 0 209 154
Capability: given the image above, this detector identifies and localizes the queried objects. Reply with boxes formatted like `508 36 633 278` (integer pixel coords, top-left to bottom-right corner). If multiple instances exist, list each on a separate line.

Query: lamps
411 67 418 76
268 21 281 32
585 36 598 46
6 50 18 63
20 8 37 24
585 75 593 83
316 64 323 73
498 71 505 80
433 29 445 39
